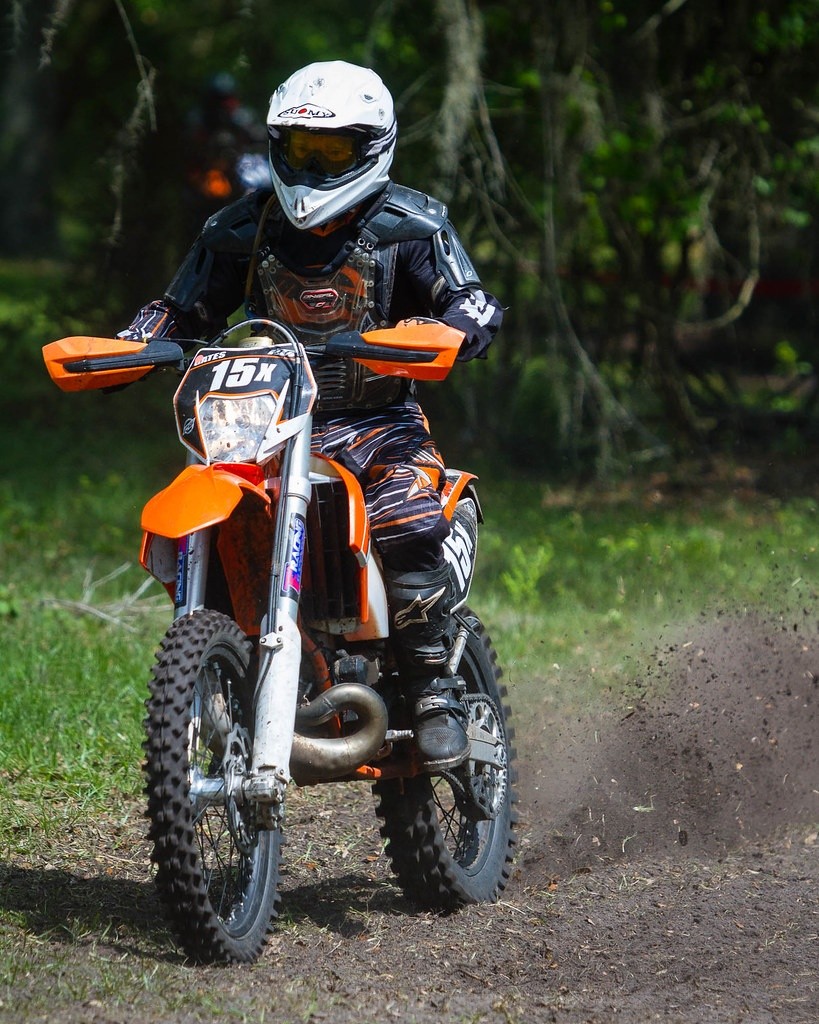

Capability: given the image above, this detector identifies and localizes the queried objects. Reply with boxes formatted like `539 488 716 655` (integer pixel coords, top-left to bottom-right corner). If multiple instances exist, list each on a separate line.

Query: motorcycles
41 325 519 970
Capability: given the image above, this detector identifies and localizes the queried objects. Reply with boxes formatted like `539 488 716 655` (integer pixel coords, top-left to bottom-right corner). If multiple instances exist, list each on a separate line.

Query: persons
110 57 510 777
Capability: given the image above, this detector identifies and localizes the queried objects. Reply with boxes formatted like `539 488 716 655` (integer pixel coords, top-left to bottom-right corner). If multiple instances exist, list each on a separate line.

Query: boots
379 564 472 773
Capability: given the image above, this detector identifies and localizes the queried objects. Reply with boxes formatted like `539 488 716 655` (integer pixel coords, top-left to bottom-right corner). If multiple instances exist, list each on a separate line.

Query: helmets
266 60 398 230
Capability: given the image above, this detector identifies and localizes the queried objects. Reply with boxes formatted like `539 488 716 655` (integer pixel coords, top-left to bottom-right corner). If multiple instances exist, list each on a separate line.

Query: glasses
280 124 367 177
